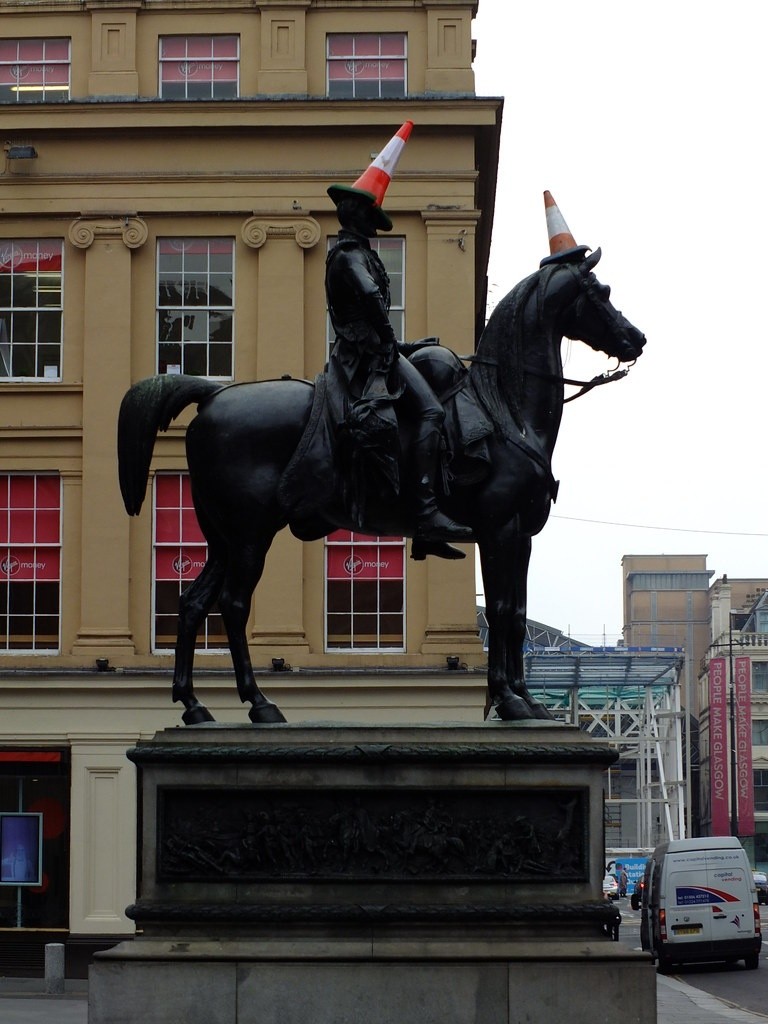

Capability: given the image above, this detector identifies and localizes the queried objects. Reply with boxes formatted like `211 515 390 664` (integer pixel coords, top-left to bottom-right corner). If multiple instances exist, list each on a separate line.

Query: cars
603 876 620 901
634 874 645 902
751 869 767 906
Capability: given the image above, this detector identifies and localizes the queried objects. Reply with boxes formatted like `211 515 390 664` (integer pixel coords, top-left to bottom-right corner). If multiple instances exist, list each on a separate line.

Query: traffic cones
539 188 595 273
327 120 416 231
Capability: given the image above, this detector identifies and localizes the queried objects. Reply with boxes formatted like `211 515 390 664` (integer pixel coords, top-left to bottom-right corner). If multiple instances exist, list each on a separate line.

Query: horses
117 248 647 722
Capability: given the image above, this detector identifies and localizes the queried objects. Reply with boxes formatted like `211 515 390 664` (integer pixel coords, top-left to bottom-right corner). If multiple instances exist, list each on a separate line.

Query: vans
630 835 763 976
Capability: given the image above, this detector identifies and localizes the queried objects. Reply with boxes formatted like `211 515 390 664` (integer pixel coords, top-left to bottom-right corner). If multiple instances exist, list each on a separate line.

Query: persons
328 187 473 561
620 873 626 898
